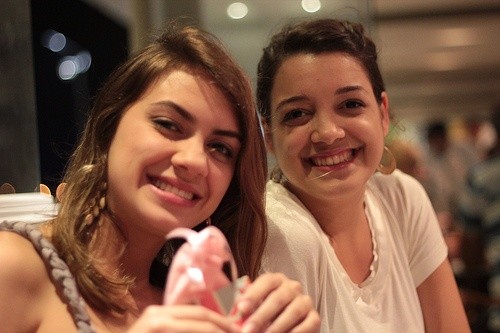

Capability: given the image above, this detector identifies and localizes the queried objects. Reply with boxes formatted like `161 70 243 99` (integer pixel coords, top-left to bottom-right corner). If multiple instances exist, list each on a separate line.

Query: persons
257 19 500 333
0 23 321 333
